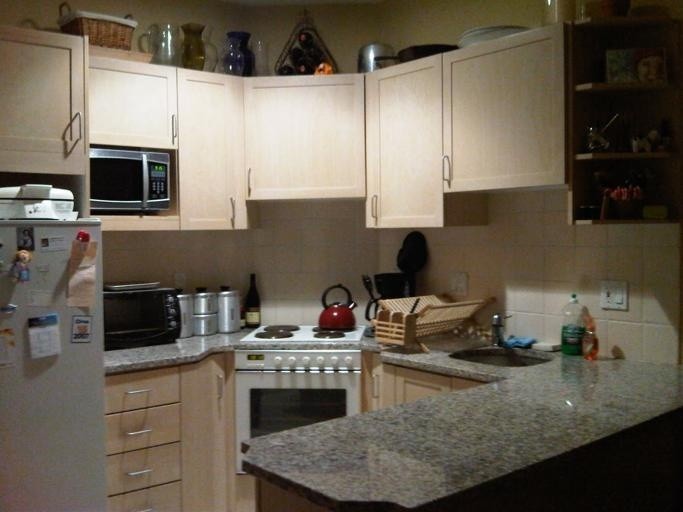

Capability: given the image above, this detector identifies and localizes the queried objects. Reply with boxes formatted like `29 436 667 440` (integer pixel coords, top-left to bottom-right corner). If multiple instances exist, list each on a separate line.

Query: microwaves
88 148 170 212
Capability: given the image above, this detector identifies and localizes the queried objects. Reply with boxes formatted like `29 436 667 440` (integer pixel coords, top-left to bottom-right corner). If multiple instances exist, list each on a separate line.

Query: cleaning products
561 294 584 359
579 302 598 359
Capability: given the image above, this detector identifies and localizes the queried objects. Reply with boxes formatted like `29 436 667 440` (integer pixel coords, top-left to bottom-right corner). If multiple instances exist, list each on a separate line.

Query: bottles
489 313 510 346
138 20 263 78
175 286 240 338
560 296 598 361
243 273 261 328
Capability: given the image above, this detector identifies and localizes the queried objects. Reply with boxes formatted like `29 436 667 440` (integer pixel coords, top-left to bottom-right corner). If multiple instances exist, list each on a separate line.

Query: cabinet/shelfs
376 362 489 413
0 25 90 178
567 15 682 224
366 53 487 230
444 21 572 194
100 353 241 512
90 55 177 152
177 66 259 233
245 72 368 203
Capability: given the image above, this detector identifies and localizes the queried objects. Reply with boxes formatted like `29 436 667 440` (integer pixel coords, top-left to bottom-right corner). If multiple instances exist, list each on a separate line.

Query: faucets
491 314 506 345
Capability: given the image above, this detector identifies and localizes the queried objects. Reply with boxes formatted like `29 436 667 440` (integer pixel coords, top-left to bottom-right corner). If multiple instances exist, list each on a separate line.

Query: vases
222 32 256 77
179 24 219 72
139 24 175 65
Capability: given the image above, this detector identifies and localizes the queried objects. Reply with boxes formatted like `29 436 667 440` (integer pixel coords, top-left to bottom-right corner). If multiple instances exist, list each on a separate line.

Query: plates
456 25 529 49
104 281 160 290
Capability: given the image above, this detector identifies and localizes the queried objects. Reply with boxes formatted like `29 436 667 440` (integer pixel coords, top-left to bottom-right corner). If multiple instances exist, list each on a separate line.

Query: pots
358 43 394 71
373 44 457 63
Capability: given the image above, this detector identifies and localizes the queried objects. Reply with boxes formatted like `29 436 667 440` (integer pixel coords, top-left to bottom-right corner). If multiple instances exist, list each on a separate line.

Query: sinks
448 347 555 368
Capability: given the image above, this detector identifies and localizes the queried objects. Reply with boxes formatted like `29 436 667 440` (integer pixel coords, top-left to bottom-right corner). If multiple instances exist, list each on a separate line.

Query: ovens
0 217 108 512
233 370 361 475
103 288 179 351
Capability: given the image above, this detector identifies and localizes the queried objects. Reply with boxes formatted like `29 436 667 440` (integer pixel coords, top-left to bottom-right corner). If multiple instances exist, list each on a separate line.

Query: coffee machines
364 231 427 323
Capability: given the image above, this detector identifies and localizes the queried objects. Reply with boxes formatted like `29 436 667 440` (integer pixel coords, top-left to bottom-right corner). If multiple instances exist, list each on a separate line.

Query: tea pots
320 285 356 330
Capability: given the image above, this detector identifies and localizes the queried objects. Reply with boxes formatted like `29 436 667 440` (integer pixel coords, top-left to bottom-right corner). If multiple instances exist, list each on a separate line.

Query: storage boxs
606 49 670 87
